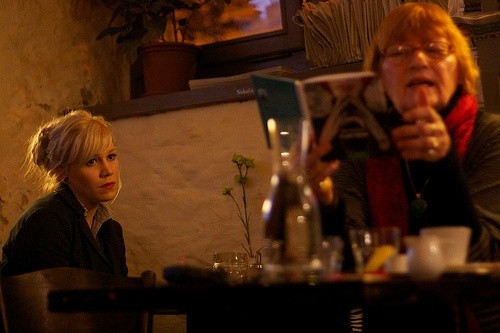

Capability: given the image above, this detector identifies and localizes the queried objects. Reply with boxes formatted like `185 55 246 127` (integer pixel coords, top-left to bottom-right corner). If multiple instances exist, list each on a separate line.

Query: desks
47 266 500 333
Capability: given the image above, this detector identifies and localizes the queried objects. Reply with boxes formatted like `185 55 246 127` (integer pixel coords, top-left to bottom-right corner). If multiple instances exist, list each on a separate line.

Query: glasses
383 41 455 65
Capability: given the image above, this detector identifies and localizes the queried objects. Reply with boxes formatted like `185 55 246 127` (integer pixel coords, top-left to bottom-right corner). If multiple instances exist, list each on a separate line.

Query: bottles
262 118 320 284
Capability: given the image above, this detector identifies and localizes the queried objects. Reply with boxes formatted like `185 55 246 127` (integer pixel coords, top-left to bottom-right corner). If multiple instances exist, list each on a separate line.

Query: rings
431 137 440 151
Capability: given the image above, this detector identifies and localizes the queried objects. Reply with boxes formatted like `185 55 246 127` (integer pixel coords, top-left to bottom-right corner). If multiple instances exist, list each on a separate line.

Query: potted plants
96 0 201 96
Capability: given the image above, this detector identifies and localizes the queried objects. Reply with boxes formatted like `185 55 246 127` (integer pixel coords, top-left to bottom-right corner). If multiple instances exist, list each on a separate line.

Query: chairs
0 266 156 333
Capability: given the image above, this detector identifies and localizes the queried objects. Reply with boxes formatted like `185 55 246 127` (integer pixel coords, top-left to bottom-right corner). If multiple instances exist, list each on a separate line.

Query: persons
264 2 500 264
0 110 131 275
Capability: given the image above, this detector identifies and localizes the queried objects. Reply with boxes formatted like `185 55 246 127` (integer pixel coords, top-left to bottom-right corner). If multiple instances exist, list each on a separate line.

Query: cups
212 252 247 283
349 226 400 284
322 235 344 280
419 225 471 277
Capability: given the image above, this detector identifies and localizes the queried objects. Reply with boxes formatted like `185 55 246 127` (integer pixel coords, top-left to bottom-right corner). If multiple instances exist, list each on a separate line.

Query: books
251 71 405 164
